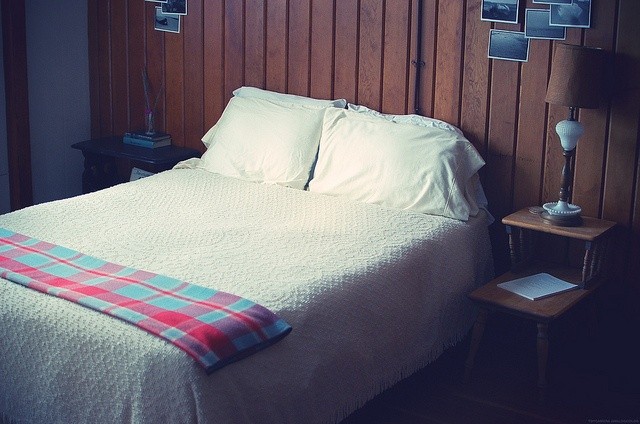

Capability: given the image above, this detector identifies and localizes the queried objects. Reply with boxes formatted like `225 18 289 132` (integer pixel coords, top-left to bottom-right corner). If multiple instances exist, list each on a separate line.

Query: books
123 130 171 149
495 273 580 300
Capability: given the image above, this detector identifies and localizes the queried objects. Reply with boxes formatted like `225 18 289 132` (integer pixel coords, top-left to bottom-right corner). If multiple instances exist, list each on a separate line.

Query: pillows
202 85 345 189
307 107 485 221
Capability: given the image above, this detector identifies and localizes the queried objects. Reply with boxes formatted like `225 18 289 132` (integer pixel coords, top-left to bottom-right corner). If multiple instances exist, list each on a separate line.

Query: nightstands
70 133 202 195
466 205 617 405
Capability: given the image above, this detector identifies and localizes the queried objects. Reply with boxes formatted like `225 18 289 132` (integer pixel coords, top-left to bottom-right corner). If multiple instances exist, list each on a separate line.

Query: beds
0 85 497 423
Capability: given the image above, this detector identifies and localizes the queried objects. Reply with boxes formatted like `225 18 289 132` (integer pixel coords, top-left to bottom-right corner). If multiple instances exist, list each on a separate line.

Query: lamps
538 44 605 227
